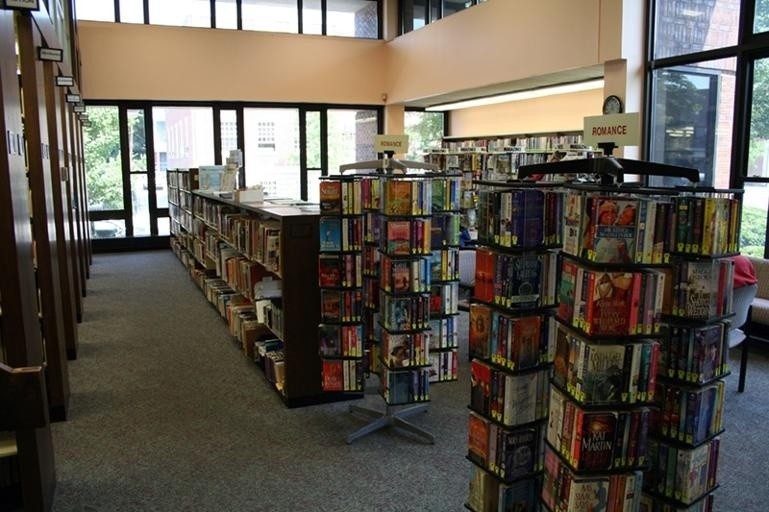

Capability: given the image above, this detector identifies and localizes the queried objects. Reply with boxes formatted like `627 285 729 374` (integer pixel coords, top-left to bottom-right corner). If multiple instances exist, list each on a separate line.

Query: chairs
457 248 476 312
743 256 769 358
727 283 758 393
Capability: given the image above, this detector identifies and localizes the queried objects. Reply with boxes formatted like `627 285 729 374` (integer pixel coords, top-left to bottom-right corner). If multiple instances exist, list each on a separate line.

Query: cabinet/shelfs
465 139 745 511
316 147 463 445
422 130 583 231
166 168 365 409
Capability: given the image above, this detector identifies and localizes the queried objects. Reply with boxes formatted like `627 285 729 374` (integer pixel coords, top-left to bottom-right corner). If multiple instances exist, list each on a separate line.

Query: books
317 176 461 406
427 136 582 242
166 170 287 402
465 188 740 511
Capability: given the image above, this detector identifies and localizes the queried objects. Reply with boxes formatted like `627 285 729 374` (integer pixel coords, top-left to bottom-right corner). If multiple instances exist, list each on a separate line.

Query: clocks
603 95 622 114
